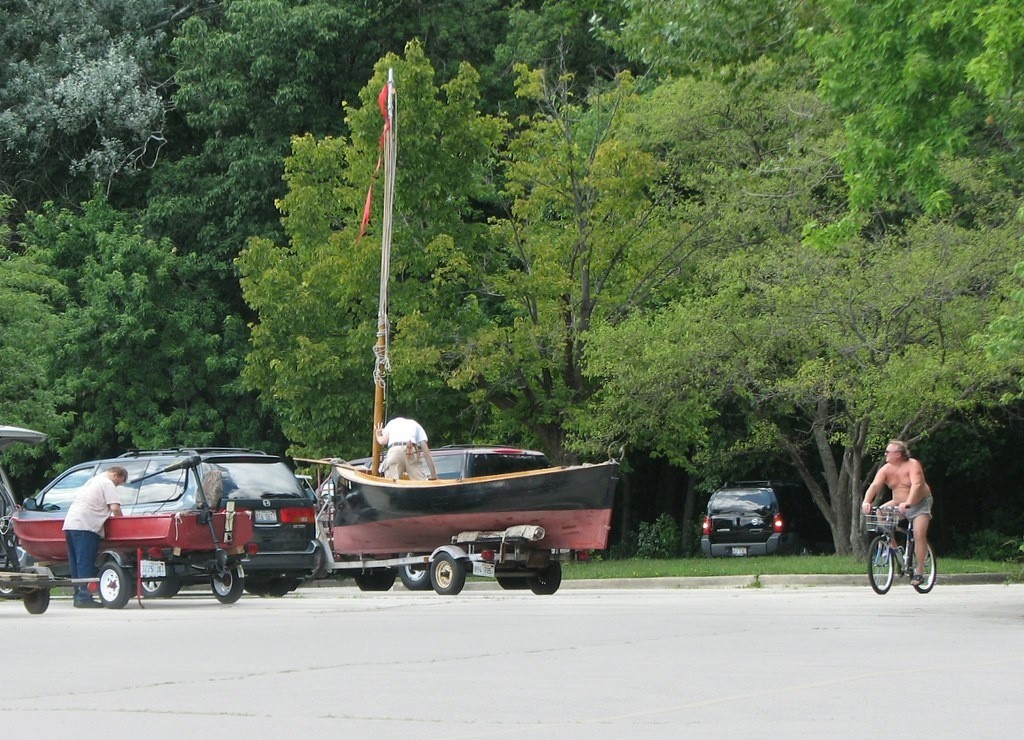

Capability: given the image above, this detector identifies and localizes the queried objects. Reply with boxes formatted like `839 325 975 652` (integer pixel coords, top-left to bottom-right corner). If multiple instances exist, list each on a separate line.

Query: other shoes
73 600 103 609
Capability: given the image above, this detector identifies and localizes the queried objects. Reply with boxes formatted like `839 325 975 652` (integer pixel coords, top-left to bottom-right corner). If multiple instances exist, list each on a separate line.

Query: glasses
885 449 903 454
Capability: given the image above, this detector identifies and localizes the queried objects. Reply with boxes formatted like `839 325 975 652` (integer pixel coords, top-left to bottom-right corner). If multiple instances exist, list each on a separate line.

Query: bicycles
862 506 937 595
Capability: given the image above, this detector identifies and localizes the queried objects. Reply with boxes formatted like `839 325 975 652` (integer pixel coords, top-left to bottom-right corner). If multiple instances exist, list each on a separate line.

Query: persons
62 465 128 608
373 416 438 481
861 440 933 585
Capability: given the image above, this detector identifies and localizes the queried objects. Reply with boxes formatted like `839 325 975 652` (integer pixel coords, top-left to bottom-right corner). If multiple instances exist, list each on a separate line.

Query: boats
331 458 621 555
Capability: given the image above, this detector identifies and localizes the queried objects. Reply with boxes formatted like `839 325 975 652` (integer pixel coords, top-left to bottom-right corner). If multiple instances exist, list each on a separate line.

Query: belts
387 442 419 449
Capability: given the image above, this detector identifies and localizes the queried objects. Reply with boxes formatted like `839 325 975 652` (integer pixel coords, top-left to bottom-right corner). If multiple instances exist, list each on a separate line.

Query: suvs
701 480 827 556
0 424 322 616
316 444 552 590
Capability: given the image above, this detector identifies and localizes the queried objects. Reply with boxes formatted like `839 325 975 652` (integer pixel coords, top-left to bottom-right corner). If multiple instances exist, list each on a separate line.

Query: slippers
909 575 926 587
